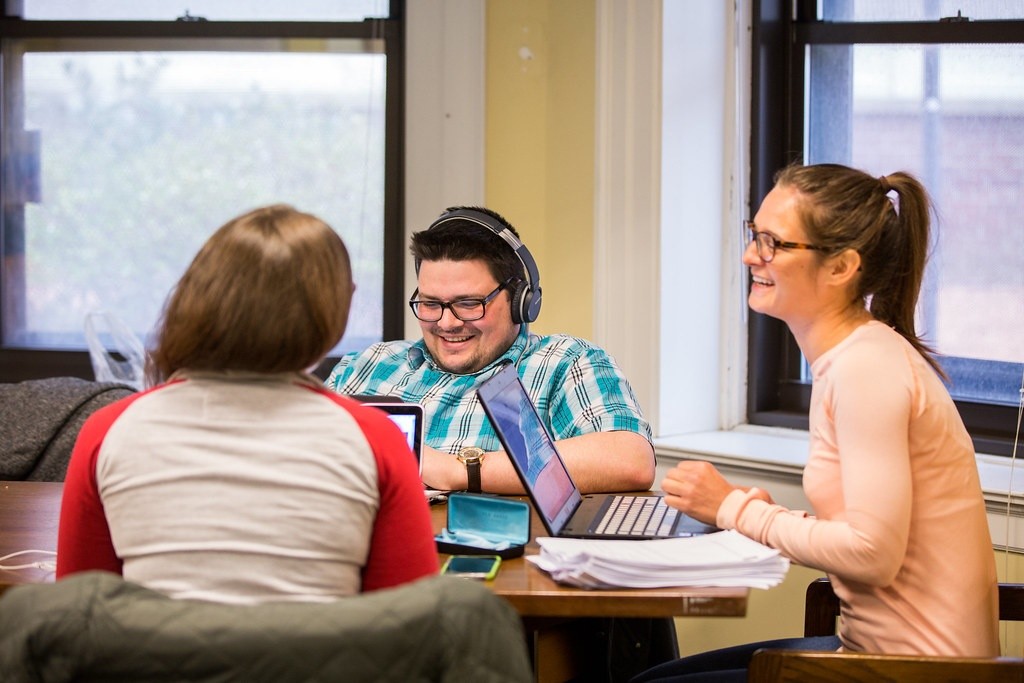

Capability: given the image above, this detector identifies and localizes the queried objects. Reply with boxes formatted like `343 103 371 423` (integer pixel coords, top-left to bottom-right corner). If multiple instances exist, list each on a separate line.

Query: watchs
456 446 485 493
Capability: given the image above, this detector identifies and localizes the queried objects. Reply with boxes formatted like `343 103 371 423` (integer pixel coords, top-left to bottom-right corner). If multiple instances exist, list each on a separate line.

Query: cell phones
438 555 501 581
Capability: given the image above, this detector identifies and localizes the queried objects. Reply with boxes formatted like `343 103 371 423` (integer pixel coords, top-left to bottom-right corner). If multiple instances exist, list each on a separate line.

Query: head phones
413 210 542 324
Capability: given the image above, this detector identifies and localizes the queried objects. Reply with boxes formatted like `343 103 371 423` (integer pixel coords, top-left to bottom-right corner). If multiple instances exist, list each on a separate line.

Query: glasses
743 219 863 271
409 276 519 322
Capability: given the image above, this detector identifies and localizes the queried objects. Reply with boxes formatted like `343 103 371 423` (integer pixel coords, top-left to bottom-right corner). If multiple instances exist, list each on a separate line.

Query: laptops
472 361 725 542
355 401 426 483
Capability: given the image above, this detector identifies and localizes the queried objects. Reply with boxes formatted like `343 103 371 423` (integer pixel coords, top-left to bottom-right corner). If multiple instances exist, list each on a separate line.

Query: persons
325 206 657 494
55 205 441 598
630 162 1001 683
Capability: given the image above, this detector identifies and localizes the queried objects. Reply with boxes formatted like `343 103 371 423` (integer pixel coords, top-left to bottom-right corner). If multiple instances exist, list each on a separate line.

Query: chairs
753 577 1024 683
0 574 526 683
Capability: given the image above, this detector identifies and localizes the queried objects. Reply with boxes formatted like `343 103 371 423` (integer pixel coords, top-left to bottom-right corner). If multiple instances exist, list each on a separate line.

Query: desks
0 478 747 683
